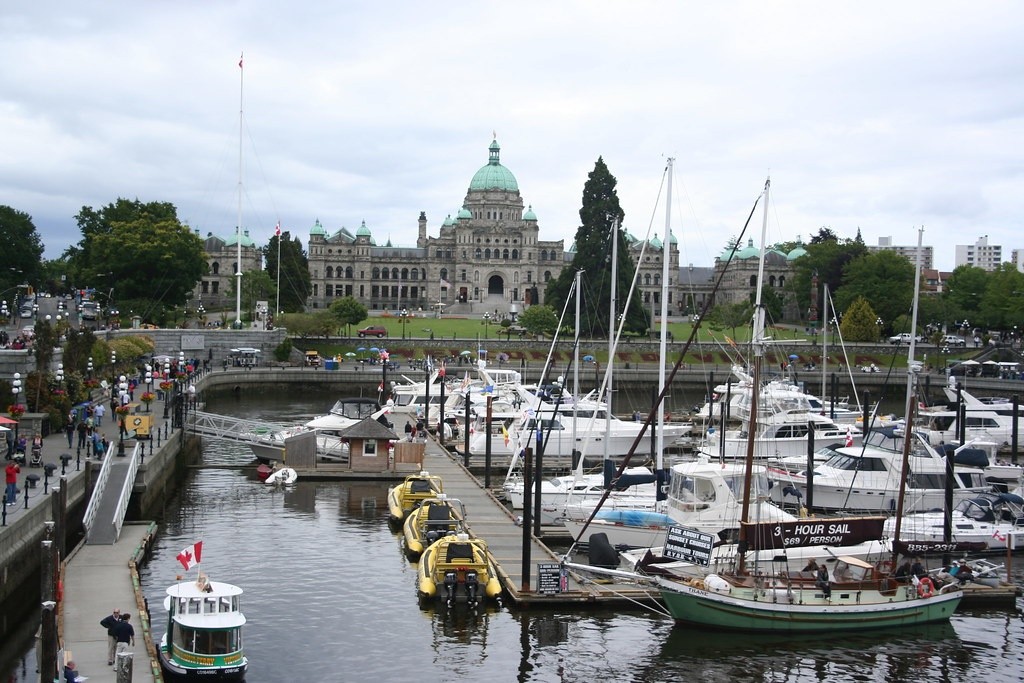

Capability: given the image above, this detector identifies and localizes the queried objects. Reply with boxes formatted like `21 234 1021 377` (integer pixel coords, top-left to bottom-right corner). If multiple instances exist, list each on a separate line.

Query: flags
501 407 535 458
442 280 451 290
845 428 853 446
275 221 282 236
378 369 493 393
992 530 1006 541
918 403 926 411
176 541 202 571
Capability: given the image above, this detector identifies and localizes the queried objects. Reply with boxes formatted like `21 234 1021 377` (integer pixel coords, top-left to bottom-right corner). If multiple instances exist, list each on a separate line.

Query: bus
229 348 260 367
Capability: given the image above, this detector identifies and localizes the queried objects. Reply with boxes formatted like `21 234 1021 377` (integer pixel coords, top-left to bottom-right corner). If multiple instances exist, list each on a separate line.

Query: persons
64 382 135 461
155 358 210 375
4 423 43 506
79 320 119 332
100 609 135 672
949 559 970 577
0 331 34 349
405 420 424 440
780 360 843 372
370 354 504 369
64 661 79 683
801 557 833 601
894 557 927 583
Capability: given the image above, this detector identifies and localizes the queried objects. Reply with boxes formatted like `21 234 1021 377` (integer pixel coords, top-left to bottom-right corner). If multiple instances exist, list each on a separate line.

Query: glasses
114 612 118 614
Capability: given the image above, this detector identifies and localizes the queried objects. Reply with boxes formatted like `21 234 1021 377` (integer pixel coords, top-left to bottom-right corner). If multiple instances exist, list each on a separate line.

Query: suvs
357 325 387 338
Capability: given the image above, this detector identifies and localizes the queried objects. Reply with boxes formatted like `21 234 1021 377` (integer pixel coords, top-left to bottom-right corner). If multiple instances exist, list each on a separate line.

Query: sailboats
650 222 965 634
247 158 1024 606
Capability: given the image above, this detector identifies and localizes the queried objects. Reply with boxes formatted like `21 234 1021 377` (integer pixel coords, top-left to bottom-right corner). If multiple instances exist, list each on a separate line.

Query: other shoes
114 668 117 671
109 662 112 665
12 502 16 505
6 503 11 506
113 661 115 663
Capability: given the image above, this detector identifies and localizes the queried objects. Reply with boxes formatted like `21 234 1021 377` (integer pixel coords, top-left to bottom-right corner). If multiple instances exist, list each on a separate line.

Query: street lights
179 351 186 393
86 356 94 380
482 311 491 338
117 375 128 458
162 358 171 419
56 363 65 391
111 350 116 388
401 308 407 339
144 365 152 413
11 372 22 449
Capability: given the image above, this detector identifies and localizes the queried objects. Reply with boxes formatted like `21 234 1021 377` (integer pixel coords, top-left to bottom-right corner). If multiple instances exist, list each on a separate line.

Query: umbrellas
460 349 509 361
947 357 997 364
0 415 19 431
788 354 799 358
344 348 387 357
583 355 593 361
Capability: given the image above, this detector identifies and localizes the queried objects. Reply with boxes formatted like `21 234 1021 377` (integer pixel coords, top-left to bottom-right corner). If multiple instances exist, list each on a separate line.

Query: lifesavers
918 578 934 598
58 580 63 602
436 423 452 442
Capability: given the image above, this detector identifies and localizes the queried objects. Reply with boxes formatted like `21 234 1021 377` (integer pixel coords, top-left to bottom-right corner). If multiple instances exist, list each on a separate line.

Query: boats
417 530 503 612
403 493 468 565
386 470 446 532
158 571 249 683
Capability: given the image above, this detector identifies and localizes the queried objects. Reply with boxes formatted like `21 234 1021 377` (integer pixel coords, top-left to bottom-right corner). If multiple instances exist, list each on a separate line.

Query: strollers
29 445 43 468
12 445 26 464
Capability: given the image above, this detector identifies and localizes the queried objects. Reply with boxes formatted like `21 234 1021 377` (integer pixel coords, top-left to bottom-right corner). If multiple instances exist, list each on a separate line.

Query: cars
20 290 99 340
